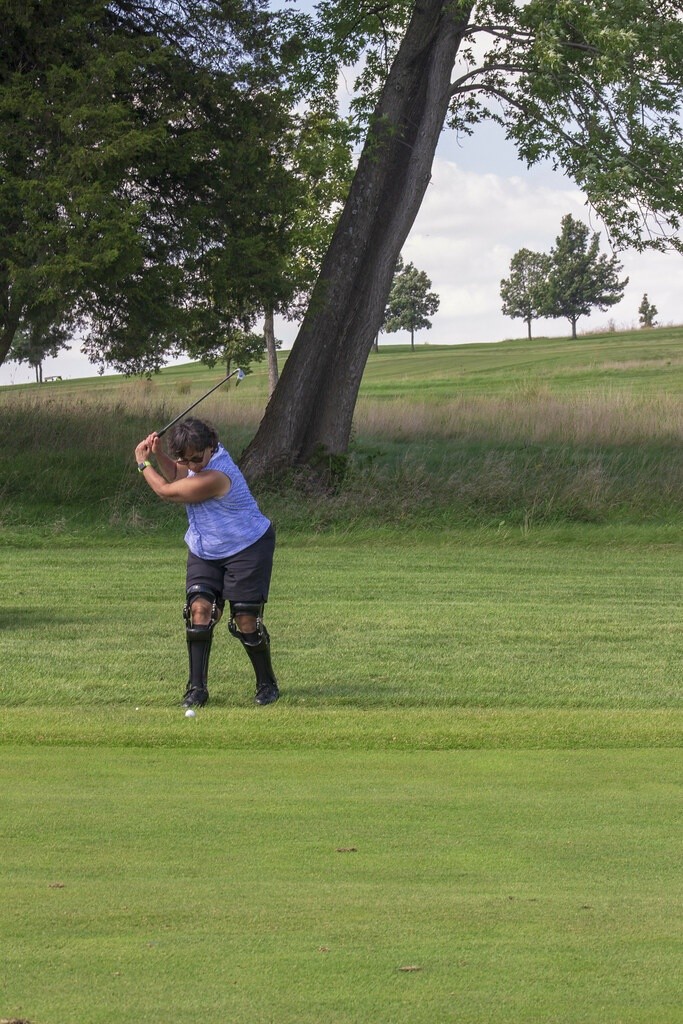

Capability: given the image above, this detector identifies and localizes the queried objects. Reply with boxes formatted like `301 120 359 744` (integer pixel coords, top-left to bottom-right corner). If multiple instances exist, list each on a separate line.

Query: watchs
137 460 150 472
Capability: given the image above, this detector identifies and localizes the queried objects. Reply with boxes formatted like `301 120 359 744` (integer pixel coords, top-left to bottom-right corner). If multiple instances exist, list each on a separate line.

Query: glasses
177 449 206 465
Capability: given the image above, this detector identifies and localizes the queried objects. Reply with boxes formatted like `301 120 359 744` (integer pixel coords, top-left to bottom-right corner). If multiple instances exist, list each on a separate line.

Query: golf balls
185 709 196 717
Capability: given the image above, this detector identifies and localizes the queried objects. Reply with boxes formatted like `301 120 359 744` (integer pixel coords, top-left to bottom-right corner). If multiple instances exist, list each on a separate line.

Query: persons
134 417 281 710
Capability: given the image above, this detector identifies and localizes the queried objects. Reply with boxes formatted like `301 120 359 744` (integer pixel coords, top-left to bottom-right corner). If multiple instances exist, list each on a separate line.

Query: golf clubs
146 366 245 455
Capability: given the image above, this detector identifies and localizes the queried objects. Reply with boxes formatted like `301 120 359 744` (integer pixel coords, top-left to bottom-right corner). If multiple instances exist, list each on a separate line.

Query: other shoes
255 687 279 705
182 691 209 707
